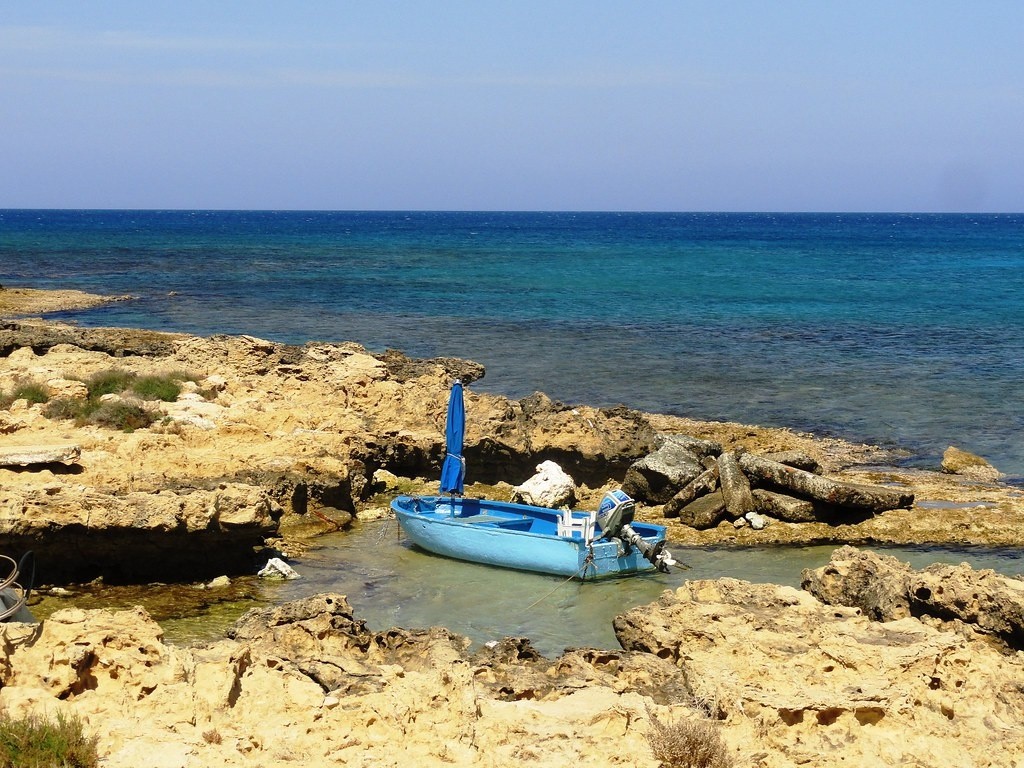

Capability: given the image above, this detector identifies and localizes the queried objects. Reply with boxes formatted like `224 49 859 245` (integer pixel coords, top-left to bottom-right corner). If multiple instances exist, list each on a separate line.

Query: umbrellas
440 379 466 517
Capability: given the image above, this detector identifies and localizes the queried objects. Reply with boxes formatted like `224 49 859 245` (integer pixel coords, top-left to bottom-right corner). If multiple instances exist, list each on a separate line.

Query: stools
555 508 597 544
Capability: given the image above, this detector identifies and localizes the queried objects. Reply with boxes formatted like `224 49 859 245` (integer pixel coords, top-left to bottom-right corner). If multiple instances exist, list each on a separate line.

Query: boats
389 377 680 582
